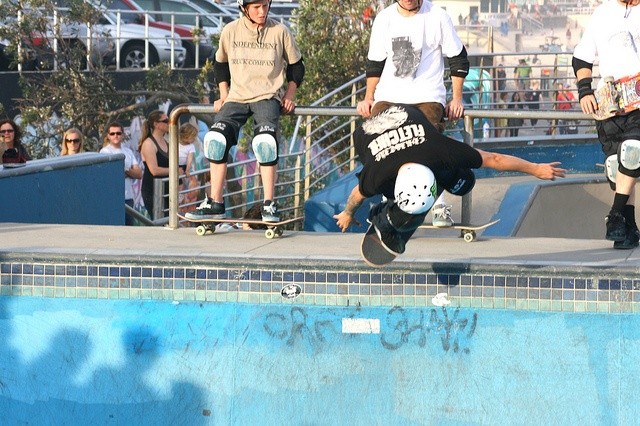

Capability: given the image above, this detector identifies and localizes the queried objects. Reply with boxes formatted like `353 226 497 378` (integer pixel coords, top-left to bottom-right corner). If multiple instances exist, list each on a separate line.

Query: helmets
394 163 438 213
237 0 273 44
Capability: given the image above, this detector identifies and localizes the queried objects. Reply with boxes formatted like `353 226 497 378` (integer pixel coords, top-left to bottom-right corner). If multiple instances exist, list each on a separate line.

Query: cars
100 1 215 67
191 0 238 25
227 1 298 32
134 1 227 67
1 0 186 68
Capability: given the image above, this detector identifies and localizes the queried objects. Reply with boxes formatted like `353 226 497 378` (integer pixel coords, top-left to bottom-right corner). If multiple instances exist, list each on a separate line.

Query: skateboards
367 218 500 242
588 72 640 120
175 213 306 238
361 193 421 268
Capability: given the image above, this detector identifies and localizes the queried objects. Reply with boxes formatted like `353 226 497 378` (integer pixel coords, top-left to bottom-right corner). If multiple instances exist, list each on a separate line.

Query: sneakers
185 193 226 219
2 147 26 168
432 205 453 227
615 219 639 249
260 200 280 222
606 207 626 241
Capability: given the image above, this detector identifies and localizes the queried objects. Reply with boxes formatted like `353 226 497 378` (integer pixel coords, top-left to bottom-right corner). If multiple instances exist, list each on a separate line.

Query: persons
358 0 470 228
497 91 507 136
570 1 640 250
524 80 544 130
184 0 305 222
99 123 143 226
59 128 85 155
550 82 578 135
176 124 197 176
140 110 171 221
238 138 248 152
0 120 33 163
508 92 523 138
497 64 507 90
566 28 571 42
188 114 198 125
532 55 540 65
514 59 534 91
331 103 568 256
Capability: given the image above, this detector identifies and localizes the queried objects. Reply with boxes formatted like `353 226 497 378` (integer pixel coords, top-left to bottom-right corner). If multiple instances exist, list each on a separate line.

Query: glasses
0 129 16 133
64 138 80 144
108 131 123 136
158 118 169 123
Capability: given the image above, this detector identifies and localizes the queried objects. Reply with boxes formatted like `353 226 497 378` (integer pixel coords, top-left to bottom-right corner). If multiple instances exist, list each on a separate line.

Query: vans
269 4 302 19
